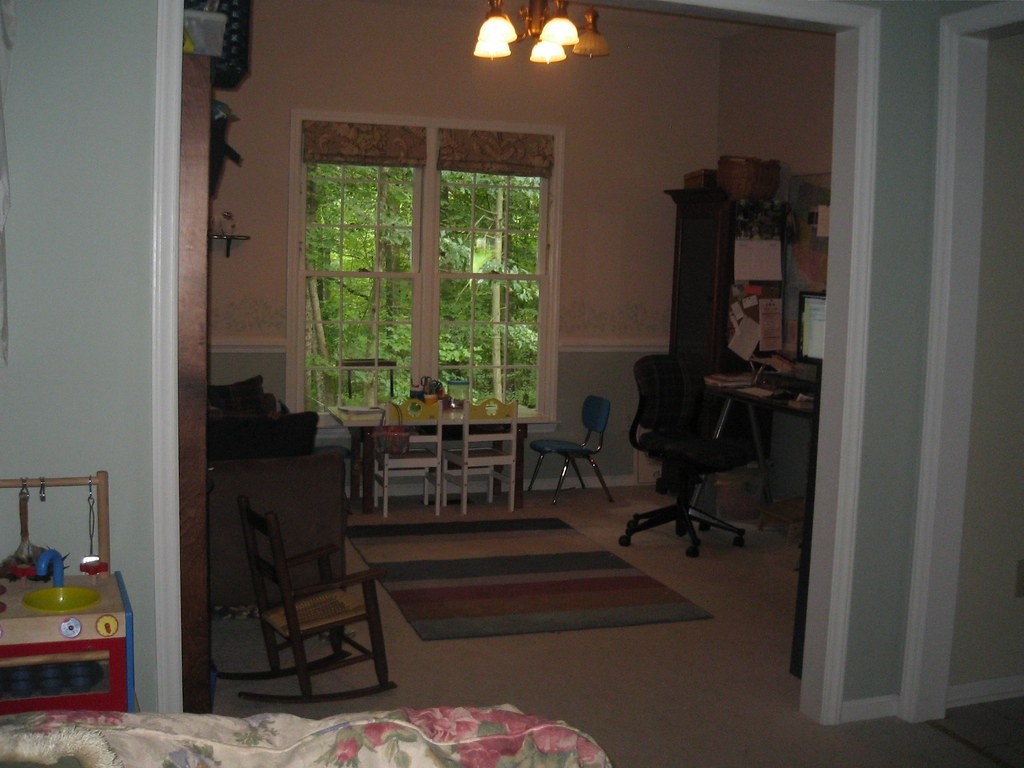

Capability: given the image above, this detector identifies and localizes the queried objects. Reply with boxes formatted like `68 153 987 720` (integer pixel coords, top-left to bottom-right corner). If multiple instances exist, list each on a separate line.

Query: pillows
207 412 320 460
223 391 276 413
208 373 263 407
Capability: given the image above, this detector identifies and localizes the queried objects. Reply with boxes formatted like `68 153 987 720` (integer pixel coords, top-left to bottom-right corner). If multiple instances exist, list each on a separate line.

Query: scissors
420 376 442 395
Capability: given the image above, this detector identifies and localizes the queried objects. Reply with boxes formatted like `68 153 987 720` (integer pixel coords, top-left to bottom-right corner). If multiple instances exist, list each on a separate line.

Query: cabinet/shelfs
655 188 783 495
0 575 135 717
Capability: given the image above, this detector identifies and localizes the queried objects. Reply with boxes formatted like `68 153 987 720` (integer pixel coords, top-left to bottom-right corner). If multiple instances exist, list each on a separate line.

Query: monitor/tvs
796 292 826 365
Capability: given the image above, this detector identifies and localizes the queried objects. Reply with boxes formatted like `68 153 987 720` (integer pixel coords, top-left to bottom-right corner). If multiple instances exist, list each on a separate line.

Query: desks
687 370 815 572
327 405 562 512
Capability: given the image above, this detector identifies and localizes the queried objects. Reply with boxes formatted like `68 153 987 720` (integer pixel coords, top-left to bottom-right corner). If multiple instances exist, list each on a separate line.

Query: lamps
473 0 610 66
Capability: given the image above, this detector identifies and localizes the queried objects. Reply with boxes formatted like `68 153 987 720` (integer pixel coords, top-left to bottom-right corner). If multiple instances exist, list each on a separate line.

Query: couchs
207 445 347 618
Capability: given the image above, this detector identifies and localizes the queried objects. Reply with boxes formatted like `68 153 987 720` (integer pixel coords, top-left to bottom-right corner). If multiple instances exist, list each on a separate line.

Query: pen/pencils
749 360 767 386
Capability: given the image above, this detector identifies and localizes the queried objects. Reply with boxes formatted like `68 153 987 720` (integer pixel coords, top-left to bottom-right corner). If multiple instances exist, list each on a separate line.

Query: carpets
345 516 715 643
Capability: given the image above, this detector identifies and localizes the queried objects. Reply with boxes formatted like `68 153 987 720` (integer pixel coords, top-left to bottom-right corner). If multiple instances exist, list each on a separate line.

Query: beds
0 703 614 768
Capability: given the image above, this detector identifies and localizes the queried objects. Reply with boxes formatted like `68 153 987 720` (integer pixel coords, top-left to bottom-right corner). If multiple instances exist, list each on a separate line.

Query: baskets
717 155 781 200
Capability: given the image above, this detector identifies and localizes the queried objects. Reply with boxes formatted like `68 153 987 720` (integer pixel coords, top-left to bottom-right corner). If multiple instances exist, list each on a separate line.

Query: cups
410 386 424 400
425 394 439 404
446 377 469 409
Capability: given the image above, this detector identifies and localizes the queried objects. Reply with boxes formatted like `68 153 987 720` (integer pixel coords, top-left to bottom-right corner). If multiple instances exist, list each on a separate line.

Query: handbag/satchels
372 402 410 457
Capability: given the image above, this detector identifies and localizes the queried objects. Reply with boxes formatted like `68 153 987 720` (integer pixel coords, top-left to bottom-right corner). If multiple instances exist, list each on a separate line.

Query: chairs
371 398 443 518
619 354 746 558
440 396 517 514
211 492 397 703
528 395 614 505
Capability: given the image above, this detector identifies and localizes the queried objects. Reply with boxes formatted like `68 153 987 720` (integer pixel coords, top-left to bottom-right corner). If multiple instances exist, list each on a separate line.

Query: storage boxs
183 10 229 58
684 169 720 190
183 0 252 91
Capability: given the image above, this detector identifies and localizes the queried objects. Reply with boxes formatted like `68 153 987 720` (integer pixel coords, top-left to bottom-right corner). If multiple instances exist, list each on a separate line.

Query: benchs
335 358 397 398
